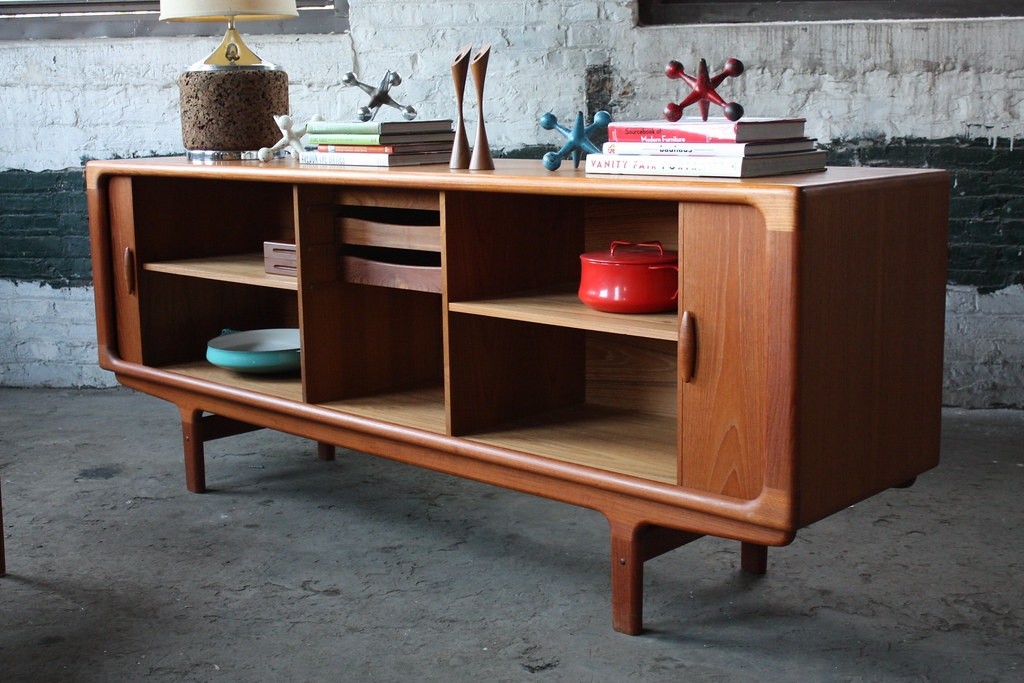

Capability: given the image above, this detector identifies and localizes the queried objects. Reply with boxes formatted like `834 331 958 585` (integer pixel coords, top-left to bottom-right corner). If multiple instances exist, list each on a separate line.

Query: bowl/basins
579 241 679 313
207 328 300 372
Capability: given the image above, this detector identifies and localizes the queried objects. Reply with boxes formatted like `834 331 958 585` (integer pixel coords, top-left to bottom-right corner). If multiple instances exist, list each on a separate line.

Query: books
299 119 456 167
584 116 828 179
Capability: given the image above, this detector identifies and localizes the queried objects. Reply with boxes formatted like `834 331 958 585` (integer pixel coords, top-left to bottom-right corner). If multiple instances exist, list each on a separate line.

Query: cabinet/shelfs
84 152 952 635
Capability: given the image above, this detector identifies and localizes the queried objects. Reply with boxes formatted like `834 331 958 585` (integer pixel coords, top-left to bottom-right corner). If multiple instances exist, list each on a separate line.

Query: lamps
158 0 299 160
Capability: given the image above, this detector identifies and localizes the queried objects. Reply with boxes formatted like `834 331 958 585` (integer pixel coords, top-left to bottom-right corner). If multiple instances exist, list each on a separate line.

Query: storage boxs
258 240 299 277
